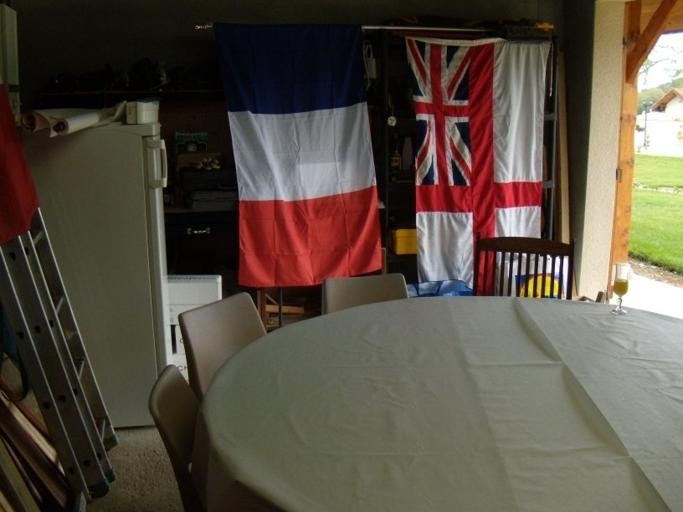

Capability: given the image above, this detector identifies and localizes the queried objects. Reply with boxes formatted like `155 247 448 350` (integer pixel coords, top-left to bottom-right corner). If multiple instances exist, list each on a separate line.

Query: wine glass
609 262 630 314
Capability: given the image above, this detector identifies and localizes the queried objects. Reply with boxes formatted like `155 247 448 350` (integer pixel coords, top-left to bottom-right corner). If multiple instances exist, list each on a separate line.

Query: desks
193 297 681 512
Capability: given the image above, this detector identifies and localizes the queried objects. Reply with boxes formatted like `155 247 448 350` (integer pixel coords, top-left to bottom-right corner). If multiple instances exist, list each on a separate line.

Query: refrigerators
18 121 172 429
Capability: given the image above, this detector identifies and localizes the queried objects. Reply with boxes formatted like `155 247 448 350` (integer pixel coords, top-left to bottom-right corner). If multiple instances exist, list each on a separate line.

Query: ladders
0 206 118 503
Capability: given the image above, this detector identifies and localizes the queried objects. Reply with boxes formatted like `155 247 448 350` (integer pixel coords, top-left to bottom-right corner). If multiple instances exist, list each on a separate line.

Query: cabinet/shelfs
165 212 236 271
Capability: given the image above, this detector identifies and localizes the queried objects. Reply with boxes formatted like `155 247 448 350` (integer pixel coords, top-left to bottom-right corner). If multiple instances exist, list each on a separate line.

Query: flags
214 23 382 289
405 34 551 291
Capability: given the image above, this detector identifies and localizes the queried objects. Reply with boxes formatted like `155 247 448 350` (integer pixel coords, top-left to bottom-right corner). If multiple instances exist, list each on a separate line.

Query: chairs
177 292 266 397
472 231 576 299
320 273 407 316
148 364 200 512
